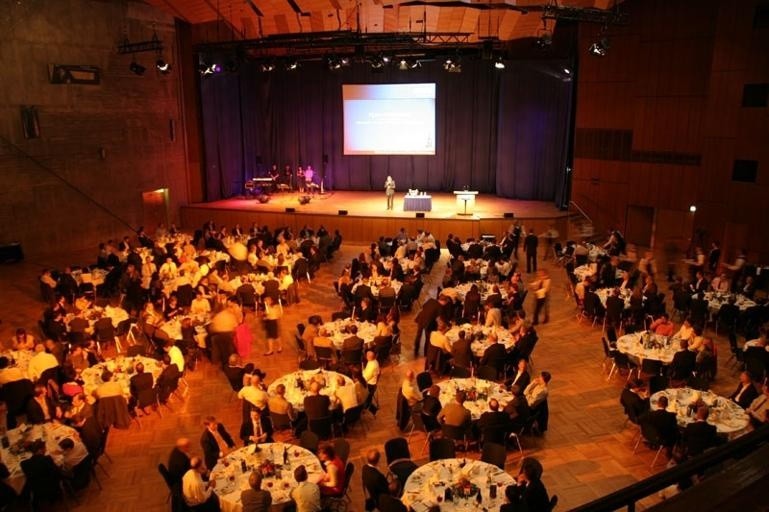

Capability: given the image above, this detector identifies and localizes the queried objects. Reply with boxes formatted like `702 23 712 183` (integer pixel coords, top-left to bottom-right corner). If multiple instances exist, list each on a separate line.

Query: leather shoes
263 351 272 356
466 444 478 452
276 347 282 353
642 441 656 450
454 445 463 451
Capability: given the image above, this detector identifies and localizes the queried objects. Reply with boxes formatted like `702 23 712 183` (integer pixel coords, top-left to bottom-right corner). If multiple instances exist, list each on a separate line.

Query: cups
739 299 744 305
478 392 487 401
497 481 502 487
1 437 10 448
687 406 694 417
712 408 720 417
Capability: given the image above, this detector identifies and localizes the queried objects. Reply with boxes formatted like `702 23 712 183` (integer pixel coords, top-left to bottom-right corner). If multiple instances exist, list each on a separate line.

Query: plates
410 500 428 512
727 422 741 427
218 485 234 495
407 482 420 493
212 471 226 479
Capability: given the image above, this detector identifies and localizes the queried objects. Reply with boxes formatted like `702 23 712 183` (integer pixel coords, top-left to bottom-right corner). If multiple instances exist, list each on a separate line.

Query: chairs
563 237 768 333
1 298 211 430
257 356 378 442
371 451 557 511
408 373 526 460
601 314 716 391
159 422 355 511
295 304 399 379
39 217 341 307
726 338 769 386
336 224 522 329
424 314 516 379
622 375 750 473
1 410 113 512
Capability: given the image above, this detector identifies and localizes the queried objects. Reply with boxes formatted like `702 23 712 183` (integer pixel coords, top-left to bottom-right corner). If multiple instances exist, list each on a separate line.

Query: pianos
252 178 273 186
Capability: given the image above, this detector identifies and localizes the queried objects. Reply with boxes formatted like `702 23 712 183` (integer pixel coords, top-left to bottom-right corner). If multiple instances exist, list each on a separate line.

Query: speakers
339 210 347 214
504 212 514 217
286 208 295 212
416 213 424 217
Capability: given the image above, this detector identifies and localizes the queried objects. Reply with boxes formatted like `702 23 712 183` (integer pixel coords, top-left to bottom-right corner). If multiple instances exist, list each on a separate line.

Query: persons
297 165 305 193
283 165 292 193
305 166 314 194
268 164 279 191
384 176 395 210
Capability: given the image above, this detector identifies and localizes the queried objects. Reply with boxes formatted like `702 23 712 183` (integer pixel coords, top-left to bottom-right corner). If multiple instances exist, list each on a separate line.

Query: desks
403 195 432 211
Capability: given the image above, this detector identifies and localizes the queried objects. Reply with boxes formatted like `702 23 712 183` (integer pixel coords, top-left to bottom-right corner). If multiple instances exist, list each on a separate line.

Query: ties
168 264 170 272
75 407 79 413
256 422 260 443
147 265 150 273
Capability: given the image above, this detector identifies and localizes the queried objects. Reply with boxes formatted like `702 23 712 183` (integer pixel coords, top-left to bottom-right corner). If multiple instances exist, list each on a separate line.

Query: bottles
443 461 498 512
675 399 679 411
640 335 643 344
42 425 48 440
240 441 289 477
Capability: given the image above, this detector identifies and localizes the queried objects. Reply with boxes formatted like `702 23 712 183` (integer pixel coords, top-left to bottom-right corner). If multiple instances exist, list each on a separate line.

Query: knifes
494 470 504 476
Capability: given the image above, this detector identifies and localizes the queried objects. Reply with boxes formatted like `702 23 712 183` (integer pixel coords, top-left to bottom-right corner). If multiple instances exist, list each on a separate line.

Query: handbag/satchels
390 336 400 355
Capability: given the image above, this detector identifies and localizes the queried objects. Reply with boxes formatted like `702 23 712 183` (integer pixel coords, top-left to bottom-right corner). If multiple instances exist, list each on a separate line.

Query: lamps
118 28 171 75
189 29 508 80
534 0 624 57
